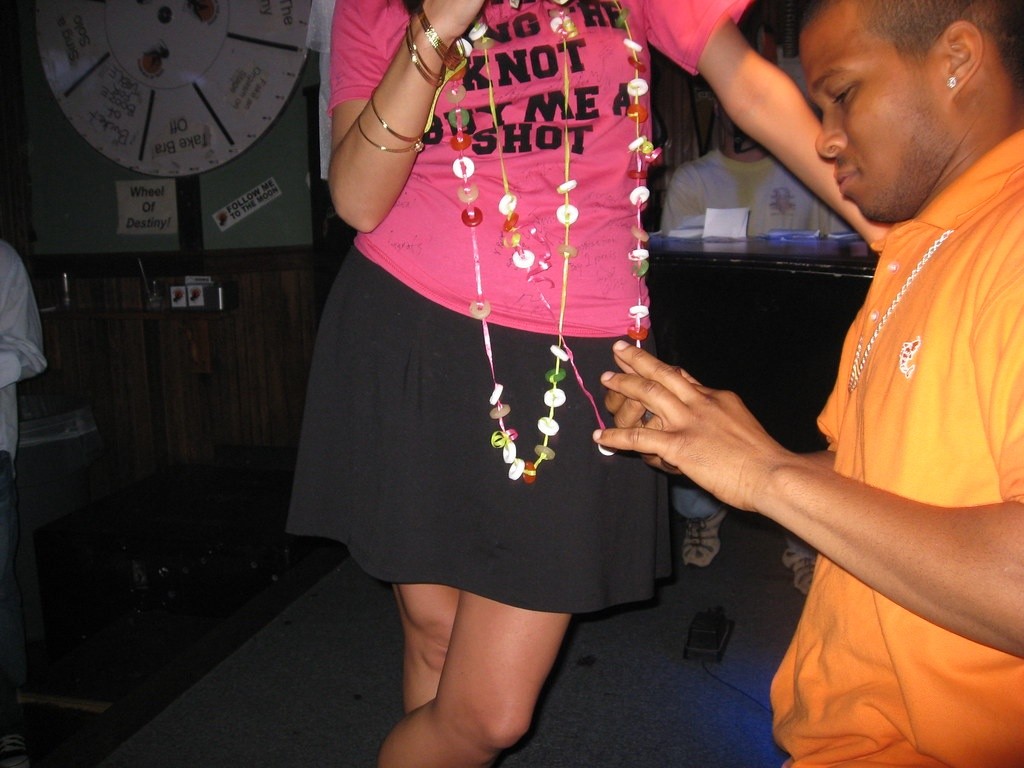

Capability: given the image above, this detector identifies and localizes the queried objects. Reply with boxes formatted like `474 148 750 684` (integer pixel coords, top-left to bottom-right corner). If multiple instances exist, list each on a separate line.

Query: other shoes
680 505 729 568
782 536 815 595
0 733 30 768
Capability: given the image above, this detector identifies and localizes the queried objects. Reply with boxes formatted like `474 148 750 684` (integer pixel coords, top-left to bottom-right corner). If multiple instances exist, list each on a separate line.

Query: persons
285 0 889 768
594 0 1024 768
0 239 49 768
659 69 852 236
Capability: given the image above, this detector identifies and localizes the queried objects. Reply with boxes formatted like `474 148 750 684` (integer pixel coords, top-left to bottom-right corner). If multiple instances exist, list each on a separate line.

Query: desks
646 241 880 532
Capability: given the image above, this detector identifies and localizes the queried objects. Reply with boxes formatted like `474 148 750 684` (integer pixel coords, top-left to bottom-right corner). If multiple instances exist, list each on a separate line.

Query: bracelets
405 6 466 86
357 88 424 153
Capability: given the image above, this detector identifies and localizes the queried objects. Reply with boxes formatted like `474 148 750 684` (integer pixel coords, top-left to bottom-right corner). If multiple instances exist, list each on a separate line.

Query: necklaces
849 230 956 391
446 0 661 485
